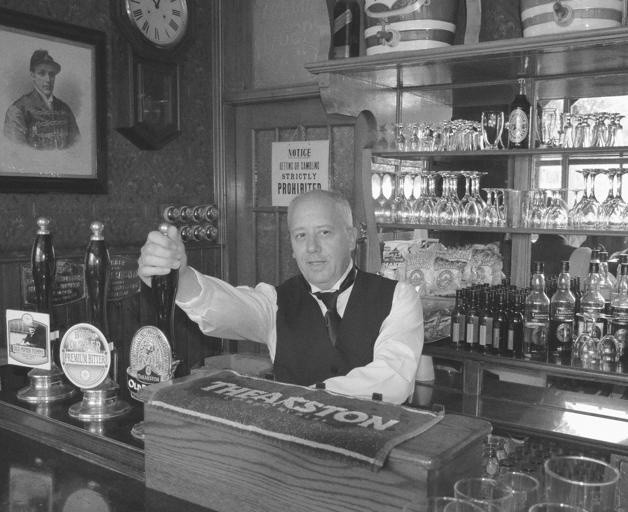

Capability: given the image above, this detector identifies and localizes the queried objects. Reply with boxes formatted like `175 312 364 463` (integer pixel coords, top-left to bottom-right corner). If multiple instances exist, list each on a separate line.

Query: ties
304 265 357 348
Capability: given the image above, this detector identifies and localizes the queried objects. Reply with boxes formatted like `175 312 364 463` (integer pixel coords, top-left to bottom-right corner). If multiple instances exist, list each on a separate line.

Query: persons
4 50 82 152
136 189 424 408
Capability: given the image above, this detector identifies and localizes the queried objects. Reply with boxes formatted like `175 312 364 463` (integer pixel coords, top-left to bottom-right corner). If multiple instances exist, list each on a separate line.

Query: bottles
332 0 360 59
447 249 628 364
509 78 532 150
478 0 523 43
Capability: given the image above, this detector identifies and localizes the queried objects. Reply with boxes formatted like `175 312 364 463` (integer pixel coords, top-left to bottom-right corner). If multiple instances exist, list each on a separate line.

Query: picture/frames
0 7 110 198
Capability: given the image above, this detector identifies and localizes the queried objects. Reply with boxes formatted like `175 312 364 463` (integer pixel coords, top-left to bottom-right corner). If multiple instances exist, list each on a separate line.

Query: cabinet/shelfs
303 24 627 393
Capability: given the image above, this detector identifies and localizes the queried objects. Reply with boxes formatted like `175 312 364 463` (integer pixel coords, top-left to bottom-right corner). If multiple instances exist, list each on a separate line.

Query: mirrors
396 69 627 314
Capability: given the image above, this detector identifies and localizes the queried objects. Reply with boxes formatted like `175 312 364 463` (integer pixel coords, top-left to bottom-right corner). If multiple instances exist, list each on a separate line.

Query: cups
460 359 484 397
415 385 434 406
415 355 437 382
401 454 620 512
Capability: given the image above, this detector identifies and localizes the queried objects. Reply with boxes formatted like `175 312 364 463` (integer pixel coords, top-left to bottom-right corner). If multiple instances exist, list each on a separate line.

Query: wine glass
372 168 628 231
535 108 626 150
378 109 508 152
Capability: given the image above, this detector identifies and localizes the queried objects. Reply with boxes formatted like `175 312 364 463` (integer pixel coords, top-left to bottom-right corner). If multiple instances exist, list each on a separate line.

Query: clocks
109 0 191 150
